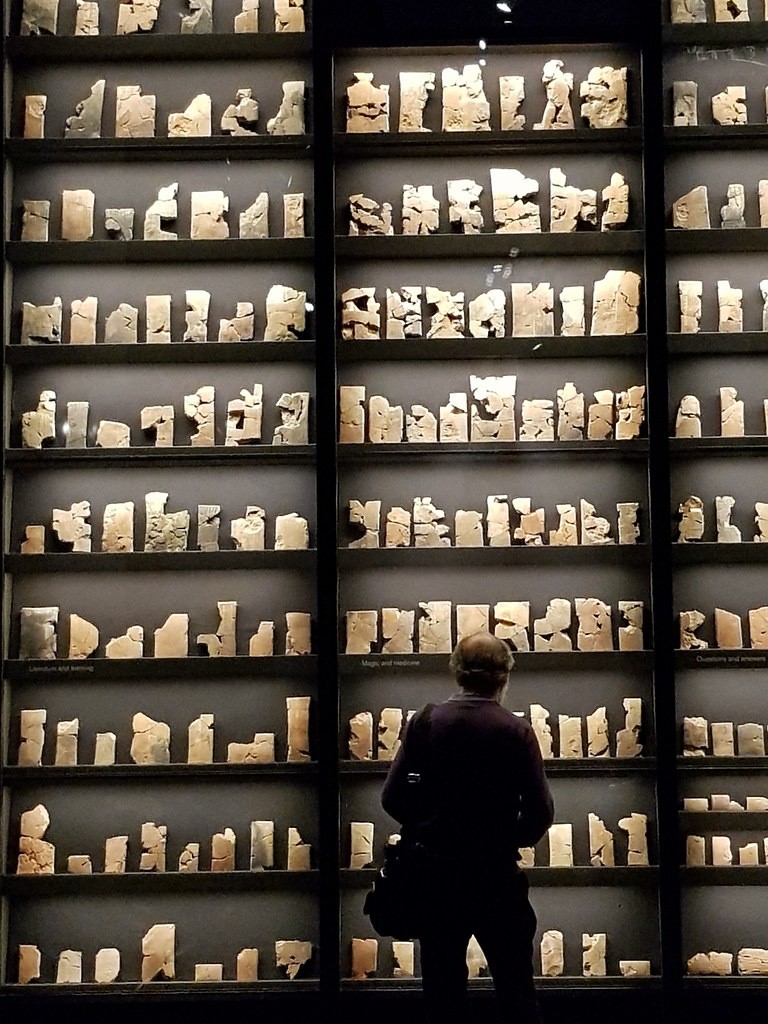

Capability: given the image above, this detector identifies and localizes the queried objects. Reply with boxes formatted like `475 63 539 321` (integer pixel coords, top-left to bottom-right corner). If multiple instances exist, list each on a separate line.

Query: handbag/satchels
362 845 428 942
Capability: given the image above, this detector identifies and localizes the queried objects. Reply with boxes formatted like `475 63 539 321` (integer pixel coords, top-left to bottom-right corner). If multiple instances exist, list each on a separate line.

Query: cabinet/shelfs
0 0 767 1024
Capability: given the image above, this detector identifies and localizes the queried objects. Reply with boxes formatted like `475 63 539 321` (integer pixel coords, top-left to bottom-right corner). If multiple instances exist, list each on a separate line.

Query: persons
380 631 556 1023
